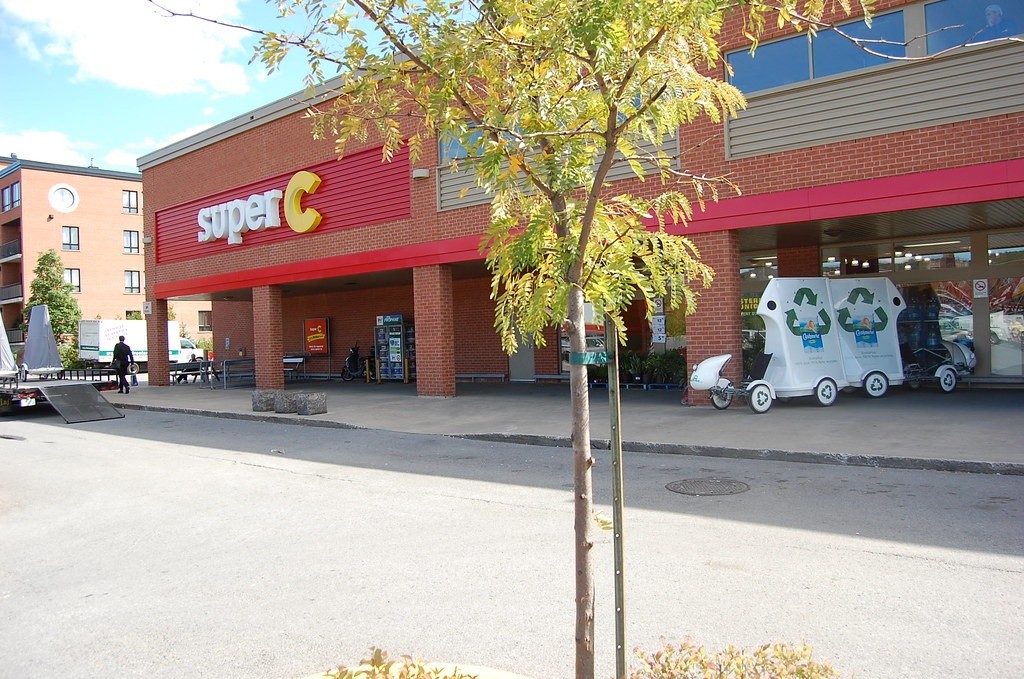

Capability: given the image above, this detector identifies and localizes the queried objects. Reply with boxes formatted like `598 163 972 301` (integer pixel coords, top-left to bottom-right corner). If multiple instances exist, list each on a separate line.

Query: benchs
62 351 312 389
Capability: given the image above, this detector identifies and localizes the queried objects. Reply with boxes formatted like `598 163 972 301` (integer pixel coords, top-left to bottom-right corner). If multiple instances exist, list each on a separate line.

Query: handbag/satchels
110 358 120 368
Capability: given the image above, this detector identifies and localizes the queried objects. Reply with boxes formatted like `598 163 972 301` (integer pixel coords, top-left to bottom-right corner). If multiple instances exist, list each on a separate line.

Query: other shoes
182 381 188 384
171 381 178 384
126 387 130 394
118 391 124 393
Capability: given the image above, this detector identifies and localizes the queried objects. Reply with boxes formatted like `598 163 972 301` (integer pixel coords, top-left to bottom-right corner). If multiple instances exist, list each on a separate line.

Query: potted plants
588 348 686 383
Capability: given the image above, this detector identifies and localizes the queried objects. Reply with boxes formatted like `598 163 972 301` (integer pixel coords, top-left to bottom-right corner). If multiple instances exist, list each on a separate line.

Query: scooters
341 341 377 382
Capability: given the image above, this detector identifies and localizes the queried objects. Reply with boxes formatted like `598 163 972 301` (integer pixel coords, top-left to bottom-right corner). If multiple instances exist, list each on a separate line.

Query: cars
560 302 605 374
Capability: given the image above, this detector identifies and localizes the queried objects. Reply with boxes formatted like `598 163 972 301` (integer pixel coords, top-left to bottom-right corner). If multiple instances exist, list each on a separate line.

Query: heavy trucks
78 318 204 375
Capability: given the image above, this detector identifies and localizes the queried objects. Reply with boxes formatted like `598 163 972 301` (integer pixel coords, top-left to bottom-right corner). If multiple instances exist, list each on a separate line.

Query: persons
112 336 134 393
171 354 200 384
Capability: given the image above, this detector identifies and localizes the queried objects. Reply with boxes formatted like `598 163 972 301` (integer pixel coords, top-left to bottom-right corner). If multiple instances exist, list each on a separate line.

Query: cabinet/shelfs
902 320 920 352
926 319 970 350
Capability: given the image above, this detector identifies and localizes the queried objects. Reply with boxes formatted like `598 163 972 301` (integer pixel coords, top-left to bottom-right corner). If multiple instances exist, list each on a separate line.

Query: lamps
143 235 154 244
750 248 929 278
412 169 429 181
